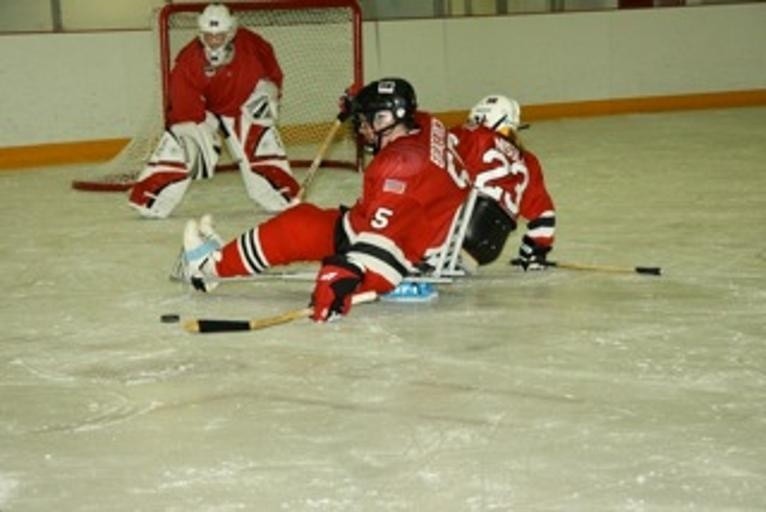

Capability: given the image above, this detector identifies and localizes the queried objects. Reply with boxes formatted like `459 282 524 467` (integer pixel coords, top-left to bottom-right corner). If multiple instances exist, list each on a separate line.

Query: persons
181 76 473 322
125 3 305 220
448 93 558 274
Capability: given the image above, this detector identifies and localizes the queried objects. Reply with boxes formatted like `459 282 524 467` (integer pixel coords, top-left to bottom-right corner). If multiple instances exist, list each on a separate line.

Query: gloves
518 238 550 268
310 258 362 321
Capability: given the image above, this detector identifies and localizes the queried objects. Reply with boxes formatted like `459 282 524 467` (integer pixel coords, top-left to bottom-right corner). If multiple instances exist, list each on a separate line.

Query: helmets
350 77 416 137
194 4 234 68
470 95 519 134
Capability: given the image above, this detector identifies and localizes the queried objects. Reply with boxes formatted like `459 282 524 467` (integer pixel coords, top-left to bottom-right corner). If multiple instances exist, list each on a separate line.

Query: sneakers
182 214 227 295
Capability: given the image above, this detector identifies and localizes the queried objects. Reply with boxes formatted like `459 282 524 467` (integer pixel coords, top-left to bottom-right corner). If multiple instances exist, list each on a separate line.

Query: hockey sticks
509 257 662 278
179 287 376 334
